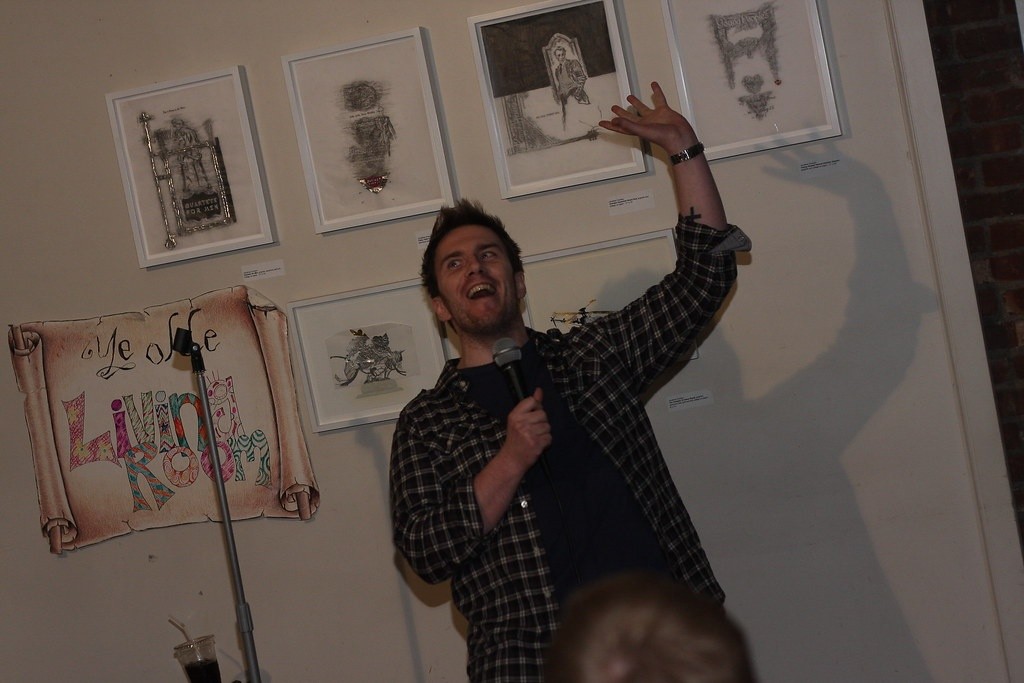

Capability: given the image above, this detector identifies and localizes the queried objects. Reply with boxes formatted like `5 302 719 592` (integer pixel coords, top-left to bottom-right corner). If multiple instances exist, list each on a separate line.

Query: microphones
492 337 530 404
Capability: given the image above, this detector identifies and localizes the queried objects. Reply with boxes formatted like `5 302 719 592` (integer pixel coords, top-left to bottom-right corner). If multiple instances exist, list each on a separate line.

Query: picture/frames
658 0 842 161
467 0 648 201
282 25 455 235
287 276 447 436
521 227 698 369
104 64 279 269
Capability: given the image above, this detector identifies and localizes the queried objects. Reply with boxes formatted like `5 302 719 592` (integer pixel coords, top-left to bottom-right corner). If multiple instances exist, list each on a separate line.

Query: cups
173 633 222 683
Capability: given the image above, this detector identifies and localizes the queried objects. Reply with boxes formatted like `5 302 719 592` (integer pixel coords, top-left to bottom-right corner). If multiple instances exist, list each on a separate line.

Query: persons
390 81 751 683
546 576 756 683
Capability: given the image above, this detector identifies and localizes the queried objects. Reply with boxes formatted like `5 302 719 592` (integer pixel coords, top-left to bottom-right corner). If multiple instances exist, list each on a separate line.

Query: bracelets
671 143 704 165
